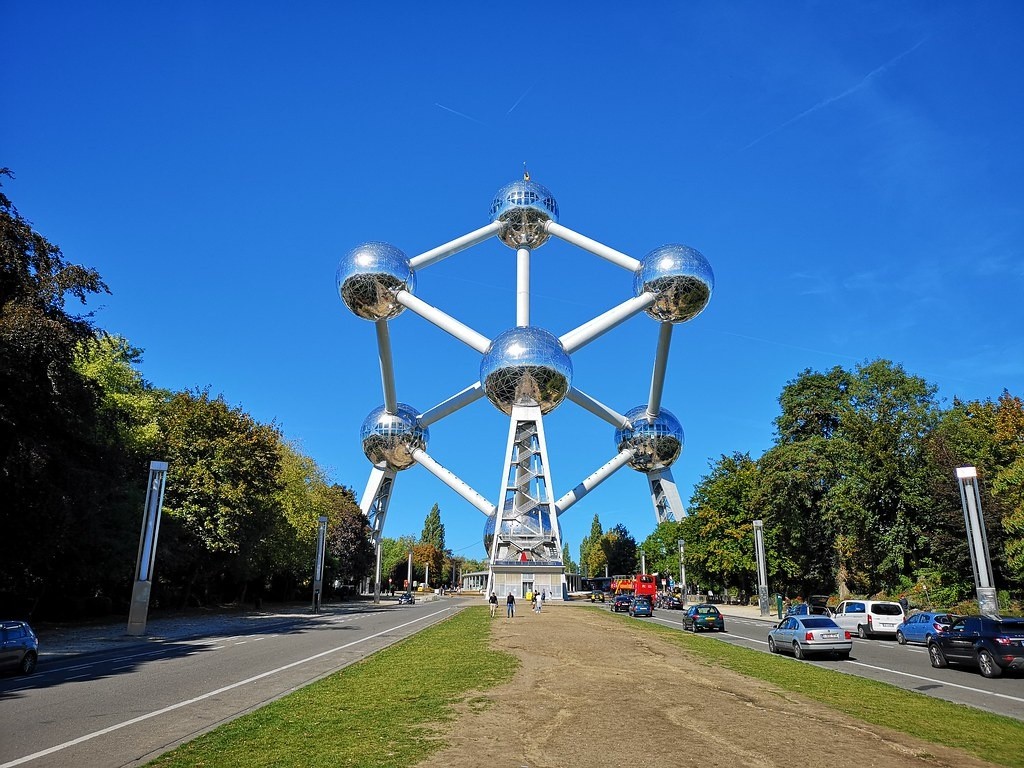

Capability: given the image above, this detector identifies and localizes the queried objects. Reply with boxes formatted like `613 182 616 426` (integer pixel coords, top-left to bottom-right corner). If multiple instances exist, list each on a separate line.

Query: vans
829 599 905 639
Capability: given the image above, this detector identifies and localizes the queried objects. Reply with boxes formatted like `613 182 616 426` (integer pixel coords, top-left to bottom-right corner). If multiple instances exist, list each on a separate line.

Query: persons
784 595 791 615
507 592 515 618
532 590 542 614
489 592 498 618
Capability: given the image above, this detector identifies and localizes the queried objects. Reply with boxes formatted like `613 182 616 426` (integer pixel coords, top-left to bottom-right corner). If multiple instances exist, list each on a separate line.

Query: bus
610 574 657 604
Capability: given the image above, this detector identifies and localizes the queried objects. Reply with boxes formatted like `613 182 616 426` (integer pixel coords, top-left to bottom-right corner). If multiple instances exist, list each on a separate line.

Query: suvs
591 590 605 603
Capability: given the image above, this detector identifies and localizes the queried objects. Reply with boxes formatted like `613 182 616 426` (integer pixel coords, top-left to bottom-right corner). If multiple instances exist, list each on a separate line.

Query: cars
608 596 631 612
682 605 724 633
896 612 966 645
0 620 39 677
629 598 653 617
399 593 415 605
784 595 833 619
768 615 853 660
656 595 684 610
928 615 1024 678
